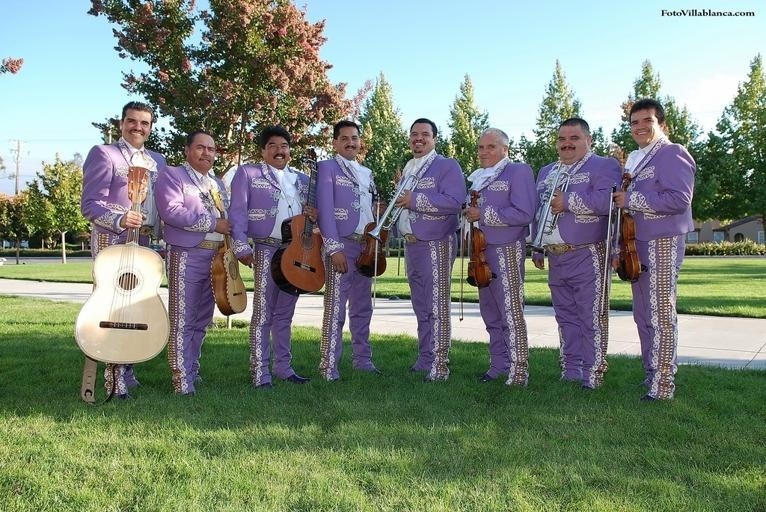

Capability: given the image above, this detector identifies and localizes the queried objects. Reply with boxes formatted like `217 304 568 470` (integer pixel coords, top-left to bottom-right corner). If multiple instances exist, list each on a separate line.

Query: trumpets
522 157 572 254
365 174 421 244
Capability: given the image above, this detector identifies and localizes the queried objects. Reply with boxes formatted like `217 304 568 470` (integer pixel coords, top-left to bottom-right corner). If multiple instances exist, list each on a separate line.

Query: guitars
74 163 173 367
208 185 249 317
270 150 326 298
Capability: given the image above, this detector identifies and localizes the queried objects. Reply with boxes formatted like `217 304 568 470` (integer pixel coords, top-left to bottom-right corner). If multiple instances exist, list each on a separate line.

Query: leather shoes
372 368 382 375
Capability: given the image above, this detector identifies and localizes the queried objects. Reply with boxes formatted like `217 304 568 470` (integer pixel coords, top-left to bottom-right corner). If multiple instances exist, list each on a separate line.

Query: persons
77 99 168 404
463 128 537 389
151 130 231 395
395 117 466 384
608 100 696 400
227 125 318 389
315 121 390 383
530 118 626 392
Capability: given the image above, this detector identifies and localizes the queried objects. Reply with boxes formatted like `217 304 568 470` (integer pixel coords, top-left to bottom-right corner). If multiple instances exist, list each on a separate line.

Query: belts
138 226 153 236
543 244 582 255
404 233 419 243
348 233 364 242
253 235 283 248
197 240 225 250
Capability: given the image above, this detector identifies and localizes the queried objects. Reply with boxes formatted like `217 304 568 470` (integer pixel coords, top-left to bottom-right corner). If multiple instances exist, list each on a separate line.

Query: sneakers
640 395 655 401
480 374 492 384
117 394 131 399
582 386 592 390
330 378 344 384
284 374 311 384
257 382 273 389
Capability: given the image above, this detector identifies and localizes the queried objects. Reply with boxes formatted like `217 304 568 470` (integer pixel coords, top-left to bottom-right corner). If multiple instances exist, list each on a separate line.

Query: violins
464 188 492 289
616 170 643 283
355 190 388 279
